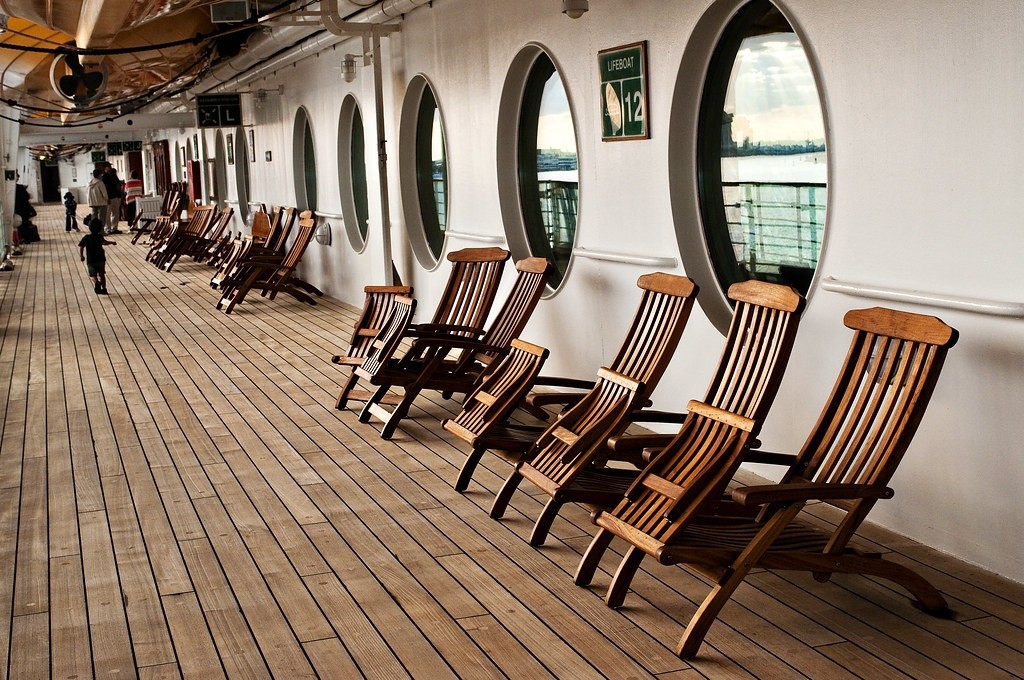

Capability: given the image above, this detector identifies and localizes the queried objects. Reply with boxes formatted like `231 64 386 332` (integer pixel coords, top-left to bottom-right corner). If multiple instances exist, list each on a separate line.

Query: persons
101 161 123 234
78 218 117 294
64 192 80 233
87 169 109 235
124 169 143 234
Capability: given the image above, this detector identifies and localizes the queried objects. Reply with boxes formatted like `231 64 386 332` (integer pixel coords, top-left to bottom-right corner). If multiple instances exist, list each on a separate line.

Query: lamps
341 48 375 83
145 128 159 140
256 82 284 108
178 118 195 134
562 0 589 19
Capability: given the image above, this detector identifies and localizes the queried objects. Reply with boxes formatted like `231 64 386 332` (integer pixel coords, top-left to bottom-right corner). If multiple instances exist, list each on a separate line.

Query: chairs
130 180 958 658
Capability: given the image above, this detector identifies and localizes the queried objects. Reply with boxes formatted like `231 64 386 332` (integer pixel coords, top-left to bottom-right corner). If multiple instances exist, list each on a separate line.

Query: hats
103 162 112 167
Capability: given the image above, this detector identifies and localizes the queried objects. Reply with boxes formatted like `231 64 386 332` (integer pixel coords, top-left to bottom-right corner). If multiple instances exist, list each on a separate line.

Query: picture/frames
226 133 234 165
249 129 255 162
597 40 650 141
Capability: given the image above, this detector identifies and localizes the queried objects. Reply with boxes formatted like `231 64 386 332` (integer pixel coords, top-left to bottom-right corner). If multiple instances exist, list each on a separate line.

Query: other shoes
67 231 70 233
101 288 108 294
94 282 101 293
128 231 133 234
114 230 122 233
76 229 79 231
